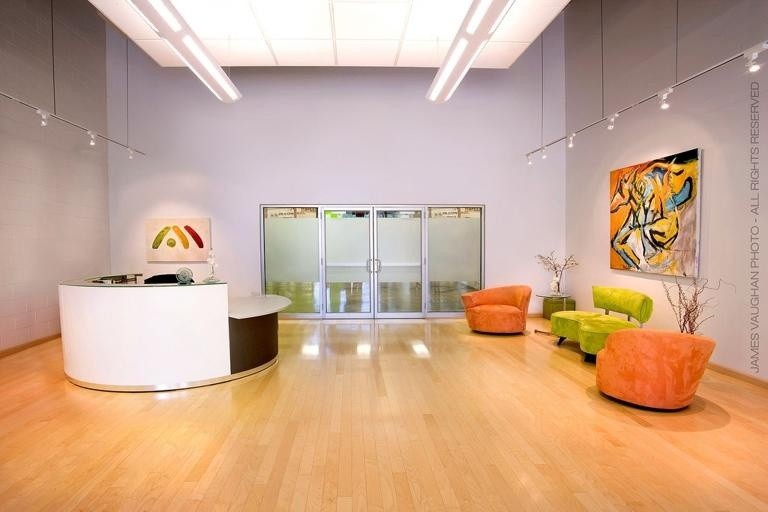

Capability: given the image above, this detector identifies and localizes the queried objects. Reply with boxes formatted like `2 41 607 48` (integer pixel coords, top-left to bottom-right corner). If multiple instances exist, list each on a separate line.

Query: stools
542 298 575 320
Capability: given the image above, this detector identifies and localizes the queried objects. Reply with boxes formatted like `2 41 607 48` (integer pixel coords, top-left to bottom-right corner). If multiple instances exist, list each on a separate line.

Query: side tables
534 294 572 337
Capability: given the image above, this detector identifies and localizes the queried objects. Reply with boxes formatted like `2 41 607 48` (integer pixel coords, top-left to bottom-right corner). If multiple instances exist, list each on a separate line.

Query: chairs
594 328 717 411
460 283 534 334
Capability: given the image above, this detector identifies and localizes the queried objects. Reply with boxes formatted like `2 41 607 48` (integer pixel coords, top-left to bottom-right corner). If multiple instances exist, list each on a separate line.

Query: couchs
550 285 654 362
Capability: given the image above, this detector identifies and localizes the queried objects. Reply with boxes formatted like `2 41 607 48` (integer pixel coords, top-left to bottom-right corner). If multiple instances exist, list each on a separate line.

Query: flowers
534 249 579 294
657 274 738 334
